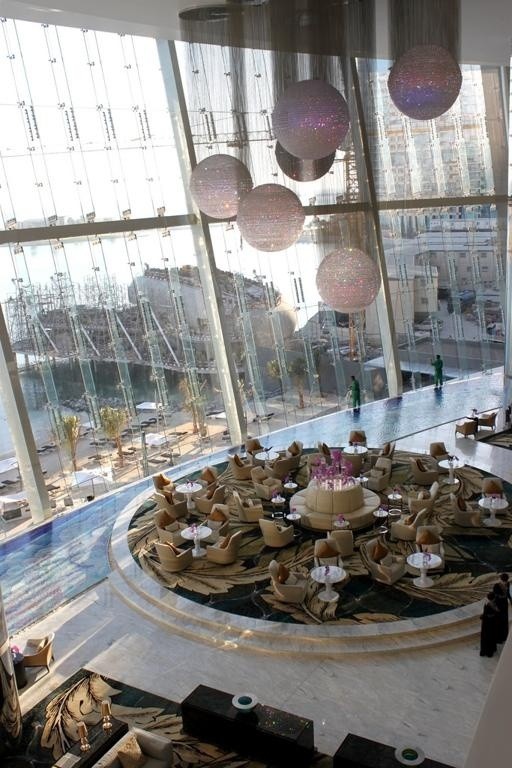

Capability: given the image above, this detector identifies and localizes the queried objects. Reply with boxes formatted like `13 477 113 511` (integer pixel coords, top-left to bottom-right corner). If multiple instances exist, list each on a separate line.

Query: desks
180 683 314 768
50 716 128 768
329 733 454 768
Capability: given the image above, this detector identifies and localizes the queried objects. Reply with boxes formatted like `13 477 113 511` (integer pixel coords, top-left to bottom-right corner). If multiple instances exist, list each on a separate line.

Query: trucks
447 290 476 313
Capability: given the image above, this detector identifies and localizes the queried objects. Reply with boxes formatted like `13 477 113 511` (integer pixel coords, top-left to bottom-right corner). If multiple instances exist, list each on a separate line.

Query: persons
492 583 507 644
349 376 360 408
500 573 512 604
479 592 497 657
430 354 443 388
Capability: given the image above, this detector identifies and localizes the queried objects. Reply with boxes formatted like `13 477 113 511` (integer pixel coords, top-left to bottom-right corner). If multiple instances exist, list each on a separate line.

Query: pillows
117 735 147 768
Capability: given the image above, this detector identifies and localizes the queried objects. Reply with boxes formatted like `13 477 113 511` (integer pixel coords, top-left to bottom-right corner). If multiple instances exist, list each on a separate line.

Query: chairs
151 408 512 605
0 417 167 522
19 632 57 673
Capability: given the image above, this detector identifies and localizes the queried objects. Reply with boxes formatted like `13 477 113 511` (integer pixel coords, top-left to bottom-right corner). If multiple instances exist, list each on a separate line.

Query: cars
414 319 444 332
310 339 383 362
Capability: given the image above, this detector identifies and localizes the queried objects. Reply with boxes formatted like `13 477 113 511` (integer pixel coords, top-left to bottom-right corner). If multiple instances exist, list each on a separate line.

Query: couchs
92 727 173 768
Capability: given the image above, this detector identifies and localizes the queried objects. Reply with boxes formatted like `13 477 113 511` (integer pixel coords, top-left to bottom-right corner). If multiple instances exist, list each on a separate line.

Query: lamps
100 700 114 738
77 720 92 751
